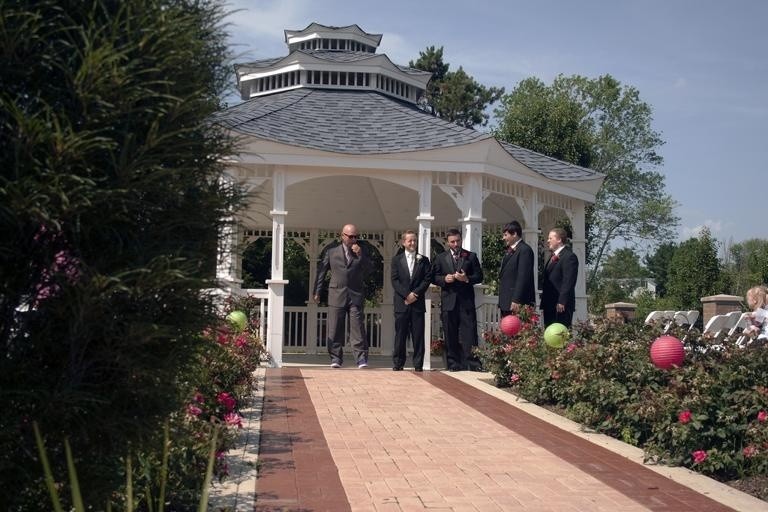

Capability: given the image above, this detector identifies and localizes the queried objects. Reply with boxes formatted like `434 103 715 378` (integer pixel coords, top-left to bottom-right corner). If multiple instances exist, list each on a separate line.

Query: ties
345 246 353 267
408 253 413 278
452 252 459 272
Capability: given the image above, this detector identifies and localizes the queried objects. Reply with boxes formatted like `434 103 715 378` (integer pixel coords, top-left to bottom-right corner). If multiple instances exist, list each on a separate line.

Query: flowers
429 338 445 357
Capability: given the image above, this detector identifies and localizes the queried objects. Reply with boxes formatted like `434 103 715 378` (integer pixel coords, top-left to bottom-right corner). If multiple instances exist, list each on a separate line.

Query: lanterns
543 321 571 349
226 310 249 332
500 314 521 336
649 334 685 370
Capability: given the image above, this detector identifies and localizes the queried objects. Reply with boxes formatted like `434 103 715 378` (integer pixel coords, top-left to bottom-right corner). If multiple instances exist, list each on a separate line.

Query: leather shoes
415 367 423 372
393 366 403 371
470 366 488 372
449 367 458 372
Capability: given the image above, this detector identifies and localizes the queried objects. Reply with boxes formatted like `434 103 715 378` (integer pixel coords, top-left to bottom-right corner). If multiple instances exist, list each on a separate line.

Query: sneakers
357 358 369 369
331 362 343 368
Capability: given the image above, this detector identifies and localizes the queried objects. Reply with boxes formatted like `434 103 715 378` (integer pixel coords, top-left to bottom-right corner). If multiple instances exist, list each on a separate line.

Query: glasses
342 232 358 240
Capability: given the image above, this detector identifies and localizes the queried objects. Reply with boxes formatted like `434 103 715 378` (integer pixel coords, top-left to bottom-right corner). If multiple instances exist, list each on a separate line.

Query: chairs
641 310 766 355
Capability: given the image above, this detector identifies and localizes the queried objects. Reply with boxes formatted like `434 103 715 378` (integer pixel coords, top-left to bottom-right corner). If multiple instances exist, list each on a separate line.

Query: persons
430 229 485 373
390 229 432 372
538 226 580 333
496 220 537 326
314 223 374 370
742 285 768 343
